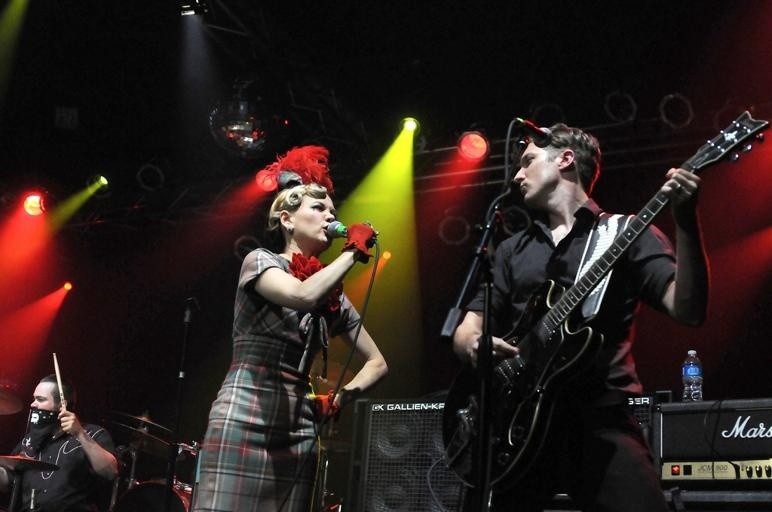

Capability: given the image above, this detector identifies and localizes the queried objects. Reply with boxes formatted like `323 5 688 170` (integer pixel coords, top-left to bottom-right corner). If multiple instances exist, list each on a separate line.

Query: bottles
682 349 704 403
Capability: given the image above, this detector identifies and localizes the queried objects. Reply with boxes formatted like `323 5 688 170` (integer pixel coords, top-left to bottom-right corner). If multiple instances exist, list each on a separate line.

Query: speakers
355 397 476 512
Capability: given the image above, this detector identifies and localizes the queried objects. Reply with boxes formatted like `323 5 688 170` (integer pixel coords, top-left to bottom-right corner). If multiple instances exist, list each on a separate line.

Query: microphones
516 117 552 148
327 221 376 243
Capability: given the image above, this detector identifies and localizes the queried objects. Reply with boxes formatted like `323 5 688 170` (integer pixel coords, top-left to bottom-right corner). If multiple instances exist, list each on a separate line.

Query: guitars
441 110 770 494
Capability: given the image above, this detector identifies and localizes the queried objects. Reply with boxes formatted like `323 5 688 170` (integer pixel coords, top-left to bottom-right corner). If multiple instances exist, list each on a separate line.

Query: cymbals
102 418 170 451
0 454 60 472
105 409 174 435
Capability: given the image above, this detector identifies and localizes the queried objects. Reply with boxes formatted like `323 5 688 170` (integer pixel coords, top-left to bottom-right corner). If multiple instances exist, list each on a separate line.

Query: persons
0 374 119 512
452 123 711 512
192 183 389 512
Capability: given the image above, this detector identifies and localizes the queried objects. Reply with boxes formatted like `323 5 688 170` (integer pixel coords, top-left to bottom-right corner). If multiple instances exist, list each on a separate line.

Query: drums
111 479 193 512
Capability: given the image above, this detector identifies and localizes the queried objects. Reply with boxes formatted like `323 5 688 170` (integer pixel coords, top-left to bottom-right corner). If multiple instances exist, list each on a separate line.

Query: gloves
342 221 378 264
311 391 341 422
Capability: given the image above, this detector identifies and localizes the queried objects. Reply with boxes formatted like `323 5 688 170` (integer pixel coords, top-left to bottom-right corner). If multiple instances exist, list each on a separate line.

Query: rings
675 184 681 192
